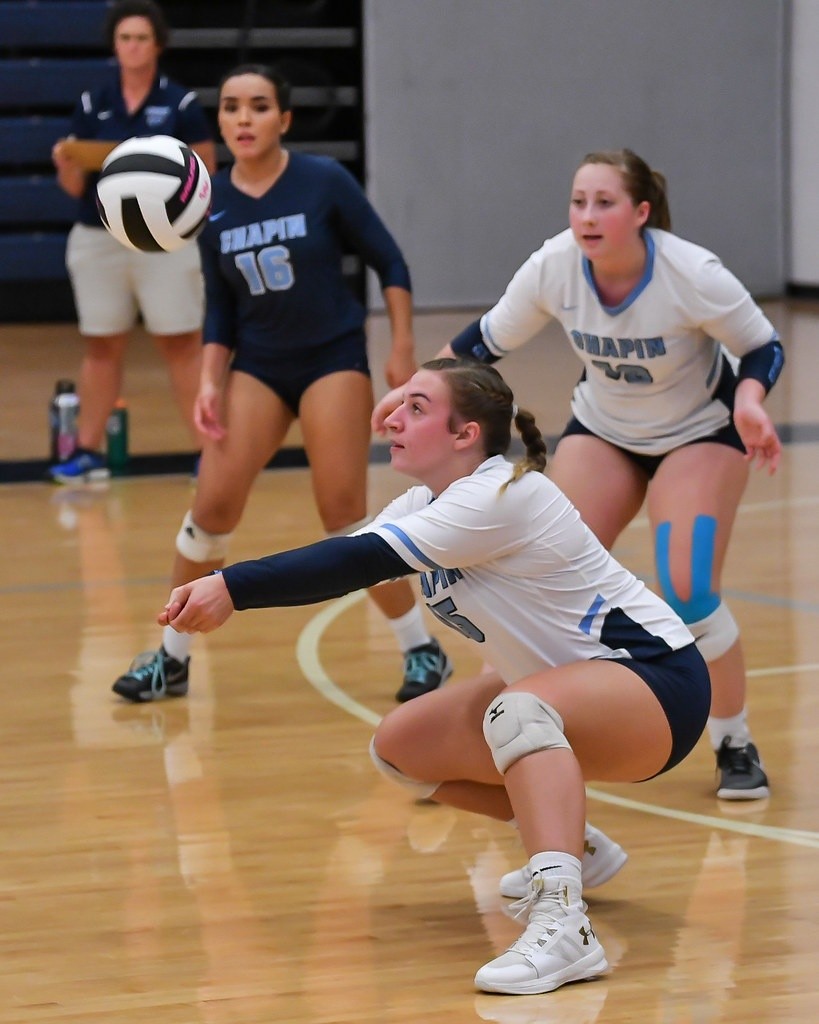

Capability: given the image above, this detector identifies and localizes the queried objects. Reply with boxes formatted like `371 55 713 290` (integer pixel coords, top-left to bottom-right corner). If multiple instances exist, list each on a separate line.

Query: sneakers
474 869 608 995
499 823 628 898
714 735 769 799
49 450 109 485
396 638 452 703
114 646 191 702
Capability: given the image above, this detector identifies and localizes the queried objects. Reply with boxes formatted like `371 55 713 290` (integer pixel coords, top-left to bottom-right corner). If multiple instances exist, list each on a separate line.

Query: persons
51 5 218 489
374 147 788 806
157 356 714 997
104 63 455 703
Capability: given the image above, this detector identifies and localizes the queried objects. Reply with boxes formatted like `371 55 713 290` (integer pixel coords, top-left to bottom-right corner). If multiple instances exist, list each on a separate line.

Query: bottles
103 399 129 464
48 380 80 461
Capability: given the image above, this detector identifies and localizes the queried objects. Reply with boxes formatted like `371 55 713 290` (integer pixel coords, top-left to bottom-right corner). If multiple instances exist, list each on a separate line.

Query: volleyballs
95 134 213 253
